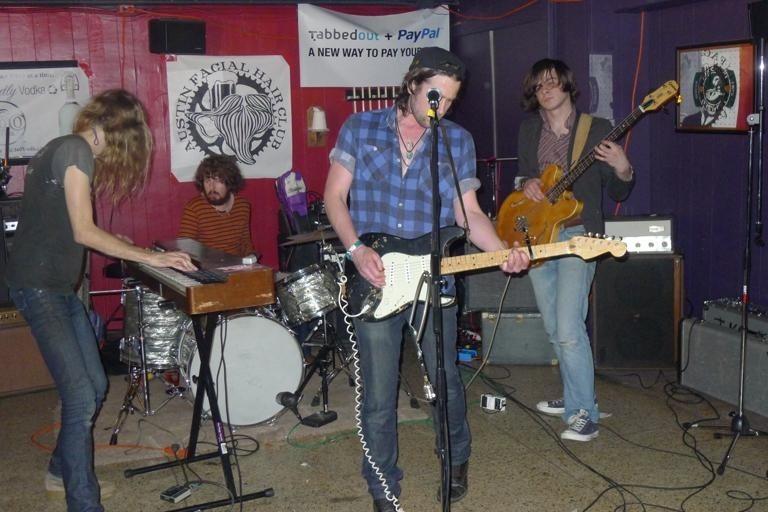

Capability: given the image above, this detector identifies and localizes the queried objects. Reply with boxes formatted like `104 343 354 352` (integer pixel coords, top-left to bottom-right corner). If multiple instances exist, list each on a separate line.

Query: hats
410 46 464 81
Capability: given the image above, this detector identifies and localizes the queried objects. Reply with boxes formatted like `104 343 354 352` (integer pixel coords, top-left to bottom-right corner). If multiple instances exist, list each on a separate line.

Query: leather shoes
373 499 398 511
438 475 468 502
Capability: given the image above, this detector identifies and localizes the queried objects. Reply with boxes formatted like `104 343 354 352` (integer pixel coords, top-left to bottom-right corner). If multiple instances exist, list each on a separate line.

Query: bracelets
345 241 363 261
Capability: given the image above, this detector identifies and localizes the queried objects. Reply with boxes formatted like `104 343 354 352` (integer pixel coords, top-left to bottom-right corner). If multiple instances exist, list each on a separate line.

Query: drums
173 311 308 429
270 262 349 325
118 279 187 375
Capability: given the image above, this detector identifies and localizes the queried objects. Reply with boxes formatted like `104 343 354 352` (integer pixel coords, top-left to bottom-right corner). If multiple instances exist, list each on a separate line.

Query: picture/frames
674 38 760 134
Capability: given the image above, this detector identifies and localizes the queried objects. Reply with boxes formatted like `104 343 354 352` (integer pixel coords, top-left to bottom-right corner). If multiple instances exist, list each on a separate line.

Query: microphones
428 88 440 112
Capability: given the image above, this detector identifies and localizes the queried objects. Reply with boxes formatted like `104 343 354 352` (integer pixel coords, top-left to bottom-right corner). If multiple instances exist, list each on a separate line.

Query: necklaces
394 120 427 161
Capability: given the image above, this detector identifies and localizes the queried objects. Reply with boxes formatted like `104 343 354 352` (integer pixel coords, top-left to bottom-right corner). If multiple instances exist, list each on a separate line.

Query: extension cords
482 394 505 411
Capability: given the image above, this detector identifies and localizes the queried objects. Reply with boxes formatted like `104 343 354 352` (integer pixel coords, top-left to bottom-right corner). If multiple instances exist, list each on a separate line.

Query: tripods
110 366 208 447
685 116 764 475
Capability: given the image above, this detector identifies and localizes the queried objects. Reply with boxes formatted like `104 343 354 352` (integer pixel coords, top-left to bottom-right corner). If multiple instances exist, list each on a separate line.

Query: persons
514 58 635 444
2 88 198 512
323 47 530 512
175 155 254 257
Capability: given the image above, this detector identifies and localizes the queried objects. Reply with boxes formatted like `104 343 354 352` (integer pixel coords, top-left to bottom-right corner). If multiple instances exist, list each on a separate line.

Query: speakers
148 18 206 56
593 254 681 371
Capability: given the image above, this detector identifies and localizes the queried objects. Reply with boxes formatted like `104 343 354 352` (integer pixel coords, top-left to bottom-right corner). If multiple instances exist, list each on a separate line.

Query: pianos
117 234 280 317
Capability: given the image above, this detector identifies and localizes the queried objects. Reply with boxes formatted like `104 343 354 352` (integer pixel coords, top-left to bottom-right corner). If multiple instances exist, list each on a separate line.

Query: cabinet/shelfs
2 309 58 397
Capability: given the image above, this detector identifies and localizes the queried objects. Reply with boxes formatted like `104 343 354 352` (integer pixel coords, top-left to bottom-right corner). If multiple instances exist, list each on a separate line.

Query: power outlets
480 394 506 412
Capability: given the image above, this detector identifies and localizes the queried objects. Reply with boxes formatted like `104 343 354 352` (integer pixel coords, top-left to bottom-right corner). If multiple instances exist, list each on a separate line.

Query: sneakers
561 410 599 442
45 471 114 499
536 397 598 416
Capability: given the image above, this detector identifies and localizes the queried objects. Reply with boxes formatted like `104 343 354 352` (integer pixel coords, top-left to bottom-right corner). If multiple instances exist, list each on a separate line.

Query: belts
564 218 584 226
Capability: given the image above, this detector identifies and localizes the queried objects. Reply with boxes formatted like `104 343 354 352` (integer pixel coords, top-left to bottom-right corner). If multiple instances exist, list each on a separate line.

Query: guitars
337 223 630 322
493 77 681 266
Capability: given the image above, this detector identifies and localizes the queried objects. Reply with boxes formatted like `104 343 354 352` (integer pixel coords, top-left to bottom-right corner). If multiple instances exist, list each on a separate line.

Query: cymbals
275 229 346 245
283 226 336 240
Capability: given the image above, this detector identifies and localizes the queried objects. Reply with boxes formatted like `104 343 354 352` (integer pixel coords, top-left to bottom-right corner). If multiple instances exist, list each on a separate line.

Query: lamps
306 104 330 147
